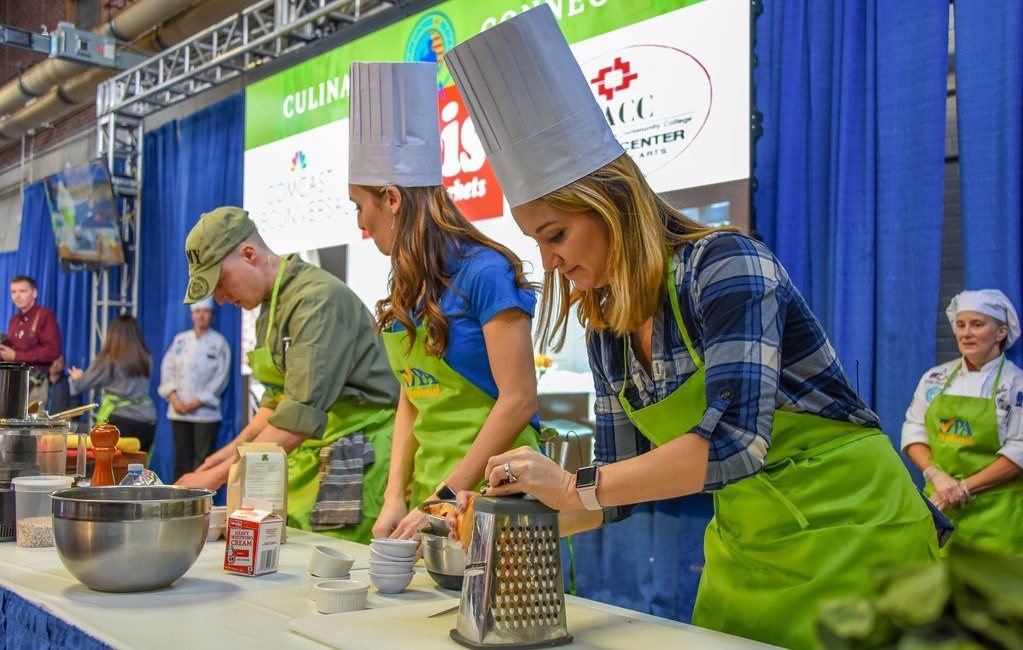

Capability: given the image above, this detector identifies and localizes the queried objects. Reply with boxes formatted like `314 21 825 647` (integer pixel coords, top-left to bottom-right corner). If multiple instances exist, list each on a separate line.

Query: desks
0 525 794 650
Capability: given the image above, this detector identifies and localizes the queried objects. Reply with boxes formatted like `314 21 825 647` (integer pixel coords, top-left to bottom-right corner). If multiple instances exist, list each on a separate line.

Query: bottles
119 463 150 485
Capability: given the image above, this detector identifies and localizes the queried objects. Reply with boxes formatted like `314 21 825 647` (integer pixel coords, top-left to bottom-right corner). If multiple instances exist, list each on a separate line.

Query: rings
504 462 517 484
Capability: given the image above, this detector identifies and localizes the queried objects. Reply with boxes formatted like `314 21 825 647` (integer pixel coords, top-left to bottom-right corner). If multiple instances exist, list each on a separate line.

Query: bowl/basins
306 570 351 601
313 579 370 614
368 499 466 594
207 506 226 542
48 484 217 593
309 545 355 578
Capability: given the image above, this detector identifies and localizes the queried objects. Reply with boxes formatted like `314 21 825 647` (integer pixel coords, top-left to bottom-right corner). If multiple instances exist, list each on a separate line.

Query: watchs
576 465 605 511
435 481 457 500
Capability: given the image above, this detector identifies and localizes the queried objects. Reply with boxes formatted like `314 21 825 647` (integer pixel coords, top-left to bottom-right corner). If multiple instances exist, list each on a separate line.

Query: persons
68 314 158 454
172 206 401 545
899 288 1022 558
0 275 85 422
348 60 542 563
158 292 232 484
444 2 941 650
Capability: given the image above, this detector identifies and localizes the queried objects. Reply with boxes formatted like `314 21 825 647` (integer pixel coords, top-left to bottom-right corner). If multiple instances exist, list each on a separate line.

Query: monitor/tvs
43 157 129 272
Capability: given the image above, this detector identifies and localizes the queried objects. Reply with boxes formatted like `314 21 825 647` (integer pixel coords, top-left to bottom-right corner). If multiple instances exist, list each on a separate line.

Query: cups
11 475 76 548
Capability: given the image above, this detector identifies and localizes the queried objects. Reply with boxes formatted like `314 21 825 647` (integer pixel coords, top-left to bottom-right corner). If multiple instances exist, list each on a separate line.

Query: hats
443 3 626 208
190 295 214 311
183 206 255 305
945 289 1021 353
348 62 443 188
